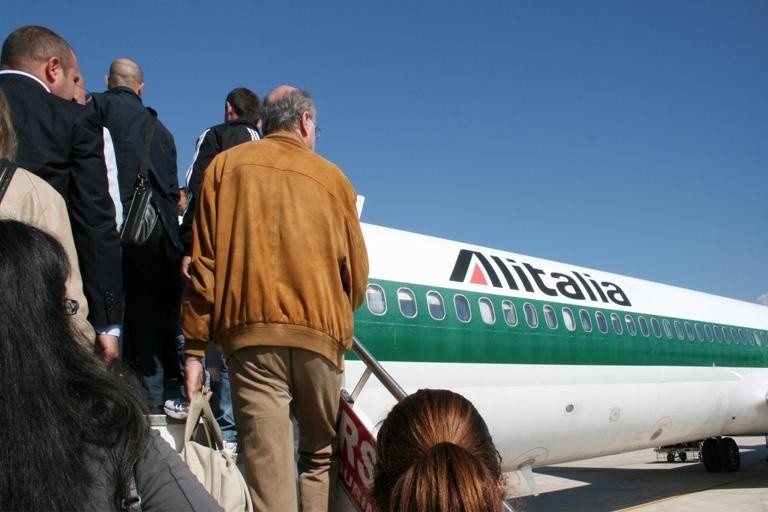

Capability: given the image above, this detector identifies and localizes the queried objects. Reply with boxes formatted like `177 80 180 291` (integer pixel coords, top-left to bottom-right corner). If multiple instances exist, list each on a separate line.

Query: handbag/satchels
120 182 186 266
174 394 256 512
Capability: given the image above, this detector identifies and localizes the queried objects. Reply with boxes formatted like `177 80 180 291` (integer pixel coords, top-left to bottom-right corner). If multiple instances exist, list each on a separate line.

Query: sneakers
162 392 208 425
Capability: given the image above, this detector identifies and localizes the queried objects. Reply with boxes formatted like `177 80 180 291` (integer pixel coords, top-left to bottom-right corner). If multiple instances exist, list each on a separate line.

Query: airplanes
142 194 768 512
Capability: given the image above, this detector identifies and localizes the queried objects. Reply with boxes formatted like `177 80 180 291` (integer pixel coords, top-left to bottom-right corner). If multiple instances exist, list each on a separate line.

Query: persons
177 185 188 225
372 384 509 511
177 82 372 512
1 24 177 415
0 215 227 511
162 85 262 463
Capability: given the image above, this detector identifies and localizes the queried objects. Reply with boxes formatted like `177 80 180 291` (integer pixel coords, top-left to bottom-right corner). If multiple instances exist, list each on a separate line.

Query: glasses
75 81 94 99
308 115 325 138
